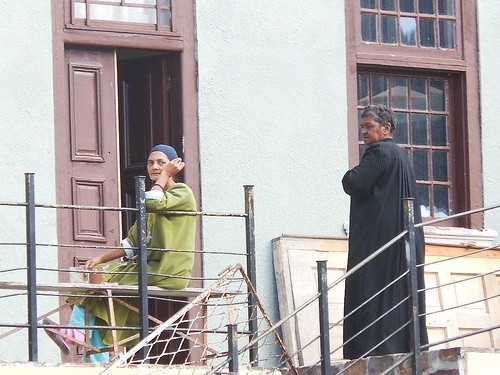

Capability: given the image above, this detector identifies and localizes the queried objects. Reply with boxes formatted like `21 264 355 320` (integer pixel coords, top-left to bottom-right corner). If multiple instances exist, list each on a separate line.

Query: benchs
0 281 243 363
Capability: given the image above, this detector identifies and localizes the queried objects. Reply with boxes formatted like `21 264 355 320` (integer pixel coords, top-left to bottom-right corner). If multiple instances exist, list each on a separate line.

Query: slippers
43 318 70 357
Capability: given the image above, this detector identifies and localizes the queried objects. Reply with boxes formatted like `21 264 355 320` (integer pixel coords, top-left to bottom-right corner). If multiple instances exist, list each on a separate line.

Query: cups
89 266 101 284
70 267 84 283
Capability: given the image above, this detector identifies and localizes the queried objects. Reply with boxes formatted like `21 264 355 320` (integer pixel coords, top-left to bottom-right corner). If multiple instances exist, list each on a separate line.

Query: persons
42 145 196 364
342 104 429 359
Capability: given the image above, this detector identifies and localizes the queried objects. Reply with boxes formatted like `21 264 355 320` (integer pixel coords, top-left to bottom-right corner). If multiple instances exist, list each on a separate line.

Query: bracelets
152 183 164 191
